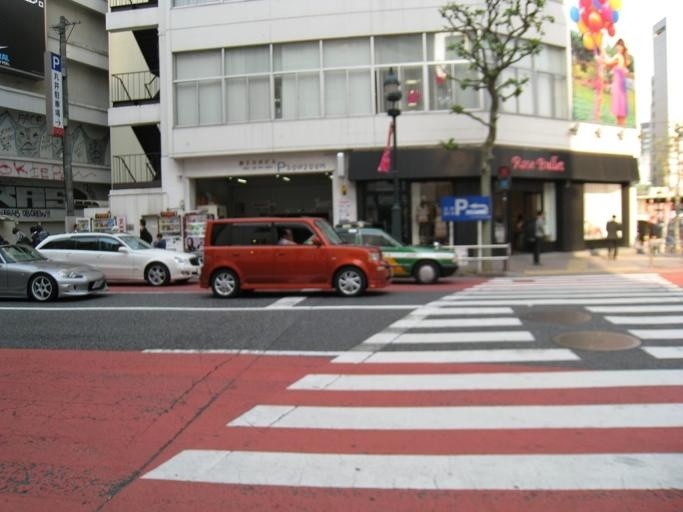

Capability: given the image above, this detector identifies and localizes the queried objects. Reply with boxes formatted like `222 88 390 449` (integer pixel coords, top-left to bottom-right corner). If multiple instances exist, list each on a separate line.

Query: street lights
382 66 404 243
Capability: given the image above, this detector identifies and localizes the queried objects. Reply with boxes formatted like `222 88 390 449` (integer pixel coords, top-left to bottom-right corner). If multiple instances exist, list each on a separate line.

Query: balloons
570 0 621 53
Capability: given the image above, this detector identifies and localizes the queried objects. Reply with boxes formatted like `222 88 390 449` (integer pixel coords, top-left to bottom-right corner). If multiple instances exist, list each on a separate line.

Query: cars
0 244 106 301
303 220 458 285
197 216 393 296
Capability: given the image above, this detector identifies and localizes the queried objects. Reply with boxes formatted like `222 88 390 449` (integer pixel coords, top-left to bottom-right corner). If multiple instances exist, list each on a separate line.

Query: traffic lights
495 162 511 191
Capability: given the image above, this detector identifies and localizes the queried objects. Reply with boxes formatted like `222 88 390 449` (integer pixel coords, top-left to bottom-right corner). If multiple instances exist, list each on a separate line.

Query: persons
512 212 526 252
186 237 199 275
152 232 166 250
413 192 432 246
71 223 81 233
37 225 50 243
634 232 648 255
531 208 548 267
277 225 308 245
600 36 631 127
137 218 153 246
29 225 38 245
11 227 32 245
604 214 622 262
663 231 675 256
648 234 661 254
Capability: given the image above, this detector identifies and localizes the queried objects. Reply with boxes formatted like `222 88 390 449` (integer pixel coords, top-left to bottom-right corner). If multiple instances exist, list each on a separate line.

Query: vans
31 232 199 287
